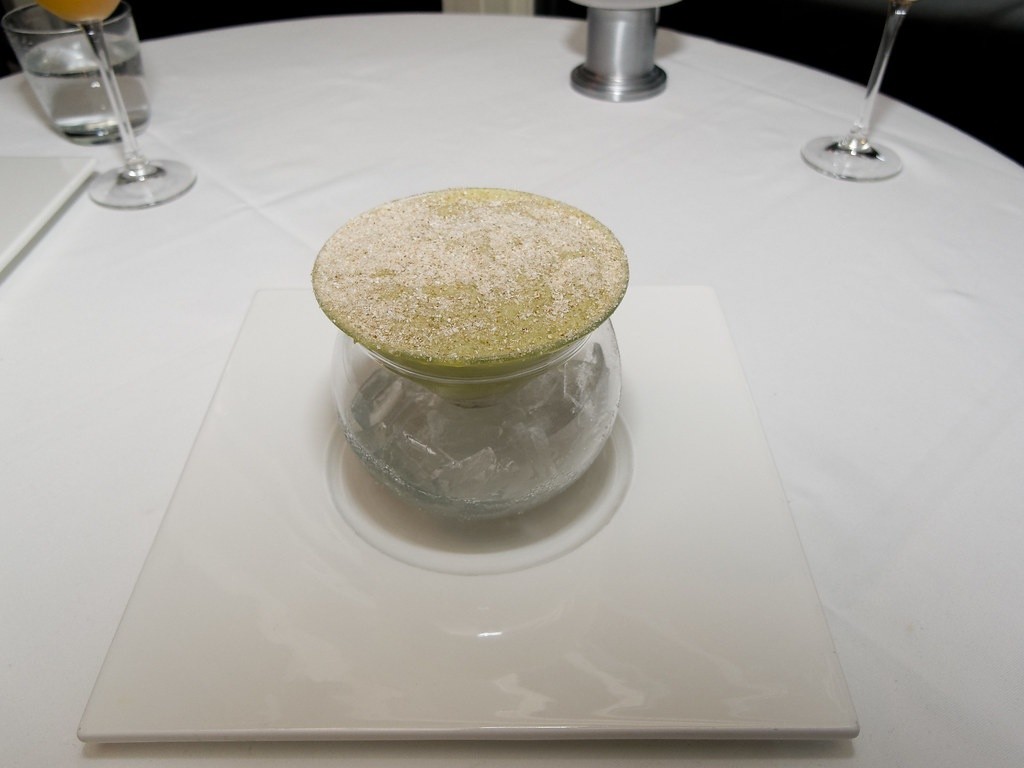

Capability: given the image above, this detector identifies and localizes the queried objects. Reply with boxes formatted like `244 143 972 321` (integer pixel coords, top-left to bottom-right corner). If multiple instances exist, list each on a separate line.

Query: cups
2 3 152 145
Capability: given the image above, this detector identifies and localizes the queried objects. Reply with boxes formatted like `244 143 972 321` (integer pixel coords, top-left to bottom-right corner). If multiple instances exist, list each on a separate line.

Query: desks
0 13 1024 768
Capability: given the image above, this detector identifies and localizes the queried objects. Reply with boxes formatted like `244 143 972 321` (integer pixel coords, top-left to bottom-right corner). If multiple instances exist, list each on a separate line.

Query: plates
0 141 97 276
74 282 862 746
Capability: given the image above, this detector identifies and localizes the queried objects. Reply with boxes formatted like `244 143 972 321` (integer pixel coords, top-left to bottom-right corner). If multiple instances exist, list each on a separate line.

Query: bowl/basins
312 186 630 530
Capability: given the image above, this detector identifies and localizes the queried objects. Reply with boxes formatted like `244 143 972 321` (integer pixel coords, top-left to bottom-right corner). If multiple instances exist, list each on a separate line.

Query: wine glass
801 0 914 182
37 0 199 210
